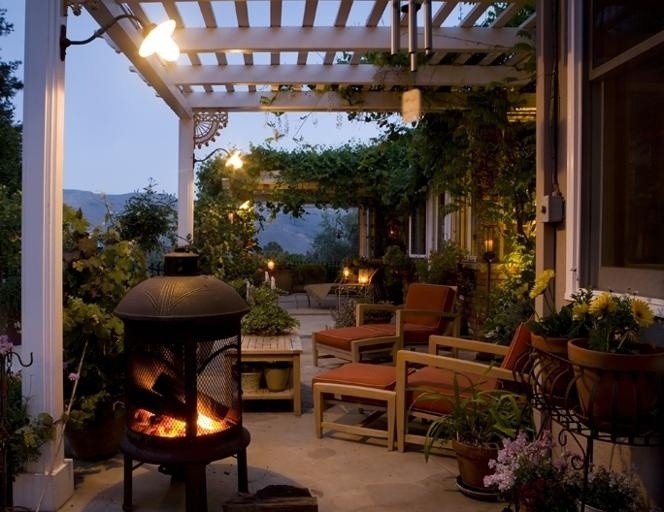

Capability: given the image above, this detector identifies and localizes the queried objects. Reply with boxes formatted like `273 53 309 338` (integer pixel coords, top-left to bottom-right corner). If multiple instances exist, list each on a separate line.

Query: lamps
57 14 180 64
192 148 243 170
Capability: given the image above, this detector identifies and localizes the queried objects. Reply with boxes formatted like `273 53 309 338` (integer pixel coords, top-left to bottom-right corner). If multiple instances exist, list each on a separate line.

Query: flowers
525 265 655 353
482 427 643 512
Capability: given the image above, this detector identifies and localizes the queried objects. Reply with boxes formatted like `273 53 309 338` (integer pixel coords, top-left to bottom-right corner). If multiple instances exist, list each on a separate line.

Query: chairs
307 280 534 454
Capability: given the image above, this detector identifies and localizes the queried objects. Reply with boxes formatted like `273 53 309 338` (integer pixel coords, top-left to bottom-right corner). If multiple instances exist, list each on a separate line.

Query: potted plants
241 280 300 336
401 358 536 493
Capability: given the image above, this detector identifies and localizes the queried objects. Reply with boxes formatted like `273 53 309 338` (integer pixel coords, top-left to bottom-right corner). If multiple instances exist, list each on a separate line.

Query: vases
529 331 663 425
571 494 637 511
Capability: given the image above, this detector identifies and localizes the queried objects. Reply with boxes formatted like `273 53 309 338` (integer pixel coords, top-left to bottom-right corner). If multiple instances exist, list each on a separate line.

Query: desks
224 331 303 418
